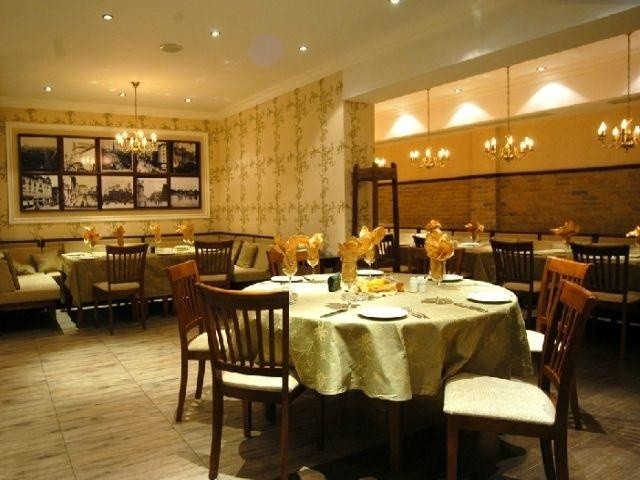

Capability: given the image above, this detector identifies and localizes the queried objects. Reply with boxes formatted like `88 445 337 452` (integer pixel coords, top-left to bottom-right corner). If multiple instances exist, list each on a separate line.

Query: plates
356 270 384 276
468 290 513 303
272 275 302 284
360 307 407 320
432 271 462 282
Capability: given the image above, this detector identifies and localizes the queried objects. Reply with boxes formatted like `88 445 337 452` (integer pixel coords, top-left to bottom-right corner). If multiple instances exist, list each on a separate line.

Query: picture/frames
3 119 213 227
15 132 203 217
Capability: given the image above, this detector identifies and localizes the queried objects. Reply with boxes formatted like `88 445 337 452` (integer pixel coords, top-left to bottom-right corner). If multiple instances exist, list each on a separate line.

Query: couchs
1 231 273 331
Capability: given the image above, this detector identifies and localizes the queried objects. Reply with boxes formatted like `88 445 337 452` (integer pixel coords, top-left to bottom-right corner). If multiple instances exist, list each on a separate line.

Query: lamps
407 88 451 169
594 30 640 153
113 80 159 155
481 65 536 162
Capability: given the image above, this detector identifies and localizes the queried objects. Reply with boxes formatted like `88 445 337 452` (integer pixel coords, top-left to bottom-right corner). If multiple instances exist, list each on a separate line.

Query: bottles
419 280 429 293
410 278 416 292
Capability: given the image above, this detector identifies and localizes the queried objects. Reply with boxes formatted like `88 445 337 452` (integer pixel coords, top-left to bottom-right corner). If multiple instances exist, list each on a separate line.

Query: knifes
454 303 487 312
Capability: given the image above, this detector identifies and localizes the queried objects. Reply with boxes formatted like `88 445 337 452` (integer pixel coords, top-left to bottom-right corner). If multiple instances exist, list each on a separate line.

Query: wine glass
432 256 445 301
287 252 298 297
81 222 197 256
366 243 377 276
341 264 358 302
308 247 319 275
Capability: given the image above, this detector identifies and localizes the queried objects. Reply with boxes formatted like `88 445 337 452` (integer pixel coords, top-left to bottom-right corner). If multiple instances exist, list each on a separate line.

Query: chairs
92 243 148 335
